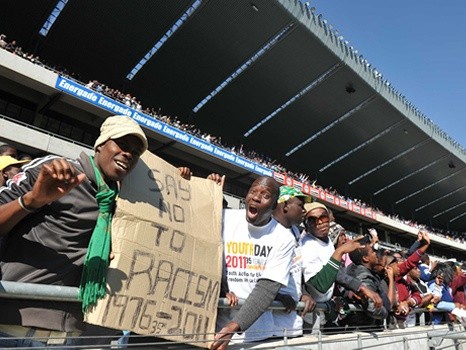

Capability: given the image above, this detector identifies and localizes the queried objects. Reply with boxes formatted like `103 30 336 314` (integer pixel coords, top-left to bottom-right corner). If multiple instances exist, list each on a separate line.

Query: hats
448 259 464 266
94 115 148 154
327 221 345 245
276 186 308 202
303 202 330 216
0 156 31 170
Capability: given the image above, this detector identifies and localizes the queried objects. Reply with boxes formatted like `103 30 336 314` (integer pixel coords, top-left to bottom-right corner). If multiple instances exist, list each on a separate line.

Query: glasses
387 256 397 265
303 213 329 228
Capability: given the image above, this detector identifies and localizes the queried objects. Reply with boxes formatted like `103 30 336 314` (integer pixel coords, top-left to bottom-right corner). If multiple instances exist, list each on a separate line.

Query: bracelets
18 192 41 214
416 249 424 256
409 298 416 306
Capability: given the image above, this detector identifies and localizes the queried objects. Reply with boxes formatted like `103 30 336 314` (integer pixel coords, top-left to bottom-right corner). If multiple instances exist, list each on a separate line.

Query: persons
0 141 33 194
299 209 466 335
206 173 297 350
0 114 194 350
271 185 317 339
325 186 466 244
142 107 224 148
298 201 367 335
231 143 320 191
0 33 76 79
85 80 142 113
